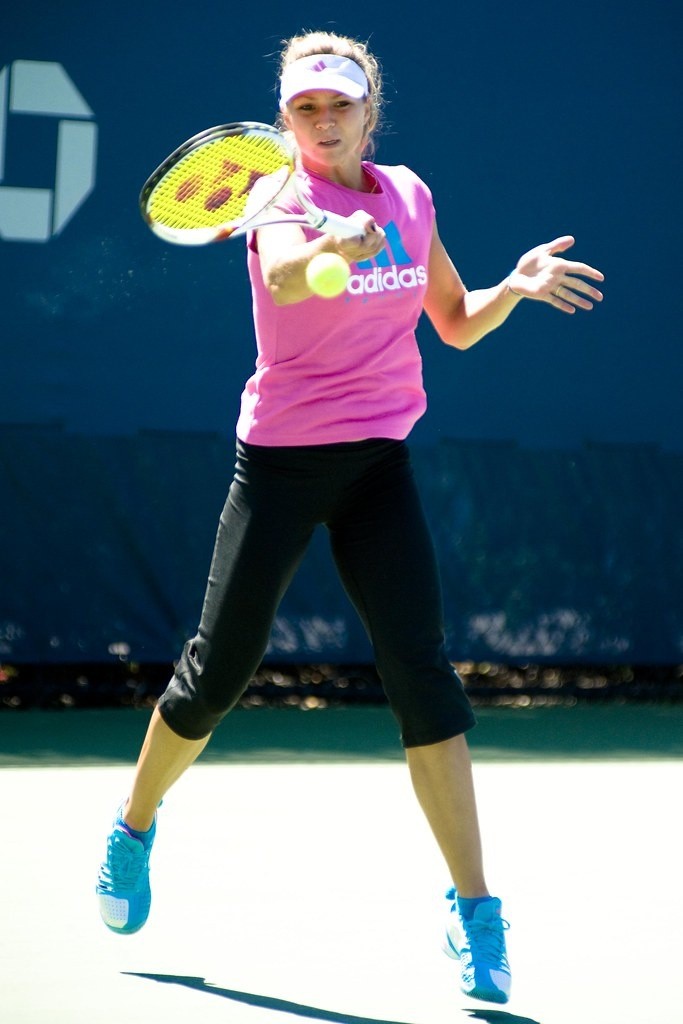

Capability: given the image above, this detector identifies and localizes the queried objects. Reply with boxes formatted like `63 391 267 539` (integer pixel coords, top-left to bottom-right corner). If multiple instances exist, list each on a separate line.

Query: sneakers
94 797 164 934
443 886 514 1004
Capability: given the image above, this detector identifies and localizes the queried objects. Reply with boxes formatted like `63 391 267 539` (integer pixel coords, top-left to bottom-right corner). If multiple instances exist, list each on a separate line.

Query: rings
556 286 562 295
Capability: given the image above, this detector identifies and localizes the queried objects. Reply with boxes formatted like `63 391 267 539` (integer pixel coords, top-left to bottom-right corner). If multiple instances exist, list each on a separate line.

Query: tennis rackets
137 120 368 247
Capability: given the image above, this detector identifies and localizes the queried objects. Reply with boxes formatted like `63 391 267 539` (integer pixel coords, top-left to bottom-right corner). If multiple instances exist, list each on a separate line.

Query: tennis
304 252 351 299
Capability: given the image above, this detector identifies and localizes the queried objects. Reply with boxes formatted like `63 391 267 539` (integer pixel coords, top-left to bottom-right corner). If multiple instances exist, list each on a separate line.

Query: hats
279 52 374 101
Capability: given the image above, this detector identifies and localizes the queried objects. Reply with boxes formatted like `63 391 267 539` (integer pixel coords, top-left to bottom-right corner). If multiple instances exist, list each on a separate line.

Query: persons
96 30 604 1005
